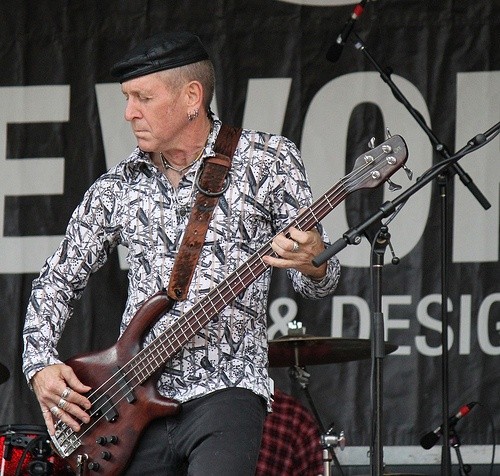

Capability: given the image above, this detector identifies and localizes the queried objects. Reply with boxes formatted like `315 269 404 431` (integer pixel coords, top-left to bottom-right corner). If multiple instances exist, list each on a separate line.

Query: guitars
45 128 409 476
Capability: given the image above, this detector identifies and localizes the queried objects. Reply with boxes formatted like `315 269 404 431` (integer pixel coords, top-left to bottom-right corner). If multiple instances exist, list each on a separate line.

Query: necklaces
159 117 215 174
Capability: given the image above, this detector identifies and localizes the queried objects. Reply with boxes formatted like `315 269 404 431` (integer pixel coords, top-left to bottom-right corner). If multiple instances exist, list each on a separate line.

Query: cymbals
0 361 11 385
266 334 399 368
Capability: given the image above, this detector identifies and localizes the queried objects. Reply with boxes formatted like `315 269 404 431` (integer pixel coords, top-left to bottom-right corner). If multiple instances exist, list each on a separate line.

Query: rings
62 387 72 398
290 240 300 252
57 398 66 408
49 406 59 415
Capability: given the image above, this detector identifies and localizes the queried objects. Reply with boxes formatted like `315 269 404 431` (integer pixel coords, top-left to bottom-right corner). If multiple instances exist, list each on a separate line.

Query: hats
108 30 208 85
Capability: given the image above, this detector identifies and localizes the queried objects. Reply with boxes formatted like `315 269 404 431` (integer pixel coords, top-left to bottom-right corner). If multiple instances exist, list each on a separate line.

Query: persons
21 30 340 476
255 388 326 476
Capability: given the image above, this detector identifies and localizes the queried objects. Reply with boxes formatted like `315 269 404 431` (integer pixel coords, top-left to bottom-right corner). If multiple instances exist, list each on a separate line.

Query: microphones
420 400 477 450
325 0 367 63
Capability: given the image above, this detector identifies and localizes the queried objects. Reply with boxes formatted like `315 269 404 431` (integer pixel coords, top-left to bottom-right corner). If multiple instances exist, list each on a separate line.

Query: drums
0 422 73 476
